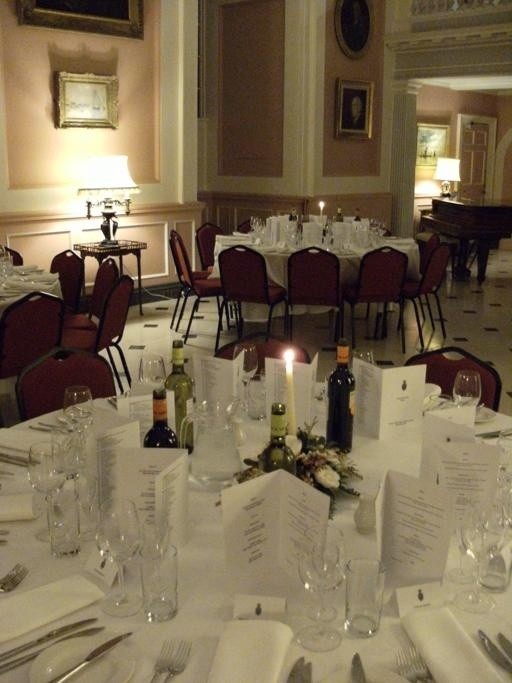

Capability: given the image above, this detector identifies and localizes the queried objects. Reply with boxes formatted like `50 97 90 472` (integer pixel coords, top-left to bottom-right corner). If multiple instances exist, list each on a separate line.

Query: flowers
284 418 365 499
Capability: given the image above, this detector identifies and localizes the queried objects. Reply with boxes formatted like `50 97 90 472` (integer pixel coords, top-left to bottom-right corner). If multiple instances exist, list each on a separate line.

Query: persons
352 98 365 128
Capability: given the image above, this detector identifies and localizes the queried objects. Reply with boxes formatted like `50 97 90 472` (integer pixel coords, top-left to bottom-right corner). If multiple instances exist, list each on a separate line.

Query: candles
281 346 299 430
316 198 326 223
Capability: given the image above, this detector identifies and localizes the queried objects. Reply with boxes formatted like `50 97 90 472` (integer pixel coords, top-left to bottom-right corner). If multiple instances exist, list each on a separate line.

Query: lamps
74 154 141 246
434 156 462 196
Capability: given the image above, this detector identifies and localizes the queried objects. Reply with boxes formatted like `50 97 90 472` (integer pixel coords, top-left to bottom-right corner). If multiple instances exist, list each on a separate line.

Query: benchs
416 230 462 279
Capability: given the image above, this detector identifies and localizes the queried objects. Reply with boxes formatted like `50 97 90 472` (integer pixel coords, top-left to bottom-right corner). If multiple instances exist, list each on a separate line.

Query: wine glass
139 354 167 390
99 496 143 617
297 544 347 650
233 342 258 410
284 222 298 250
357 218 385 254
457 426 512 616
428 368 483 419
248 216 266 247
49 425 89 499
299 524 344 622
28 440 66 541
0 246 13 292
62 383 92 460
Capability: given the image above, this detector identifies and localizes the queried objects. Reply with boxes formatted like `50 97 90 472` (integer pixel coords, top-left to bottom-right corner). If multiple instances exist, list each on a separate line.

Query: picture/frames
335 76 375 140
415 120 452 170
14 0 145 40
53 68 121 129
334 1 375 60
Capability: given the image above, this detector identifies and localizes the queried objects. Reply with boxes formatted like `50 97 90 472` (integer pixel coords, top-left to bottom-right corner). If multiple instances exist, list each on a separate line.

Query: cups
350 346 375 364
139 541 178 622
346 556 387 636
43 490 81 557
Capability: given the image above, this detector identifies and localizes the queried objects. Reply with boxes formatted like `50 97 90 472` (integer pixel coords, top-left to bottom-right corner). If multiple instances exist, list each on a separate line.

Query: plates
27 635 134 682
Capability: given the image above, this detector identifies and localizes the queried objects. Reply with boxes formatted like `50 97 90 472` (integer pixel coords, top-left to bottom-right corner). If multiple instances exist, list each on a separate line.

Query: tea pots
178 395 245 486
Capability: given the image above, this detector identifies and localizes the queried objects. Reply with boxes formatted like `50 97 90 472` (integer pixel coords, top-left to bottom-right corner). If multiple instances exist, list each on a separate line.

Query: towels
398 603 505 681
1 573 106 648
206 616 297 681
0 489 34 521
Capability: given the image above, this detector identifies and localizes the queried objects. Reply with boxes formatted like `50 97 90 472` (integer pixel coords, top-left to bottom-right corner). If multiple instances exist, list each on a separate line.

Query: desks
73 238 146 316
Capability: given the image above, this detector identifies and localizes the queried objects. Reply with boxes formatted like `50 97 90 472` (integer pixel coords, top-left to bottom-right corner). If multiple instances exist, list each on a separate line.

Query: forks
150 636 174 680
163 640 194 681
1 564 28 593
396 647 421 682
408 646 431 681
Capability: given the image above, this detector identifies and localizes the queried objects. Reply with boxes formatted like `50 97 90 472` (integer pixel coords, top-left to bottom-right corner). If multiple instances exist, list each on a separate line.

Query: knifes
0 616 98 661
495 631 512 658
350 652 365 681
47 631 134 682
0 624 105 674
287 657 313 681
477 629 512 672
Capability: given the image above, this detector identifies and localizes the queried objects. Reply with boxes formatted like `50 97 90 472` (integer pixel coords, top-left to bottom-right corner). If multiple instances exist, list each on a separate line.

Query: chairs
212 330 314 373
13 346 115 422
403 345 502 413
0 242 135 394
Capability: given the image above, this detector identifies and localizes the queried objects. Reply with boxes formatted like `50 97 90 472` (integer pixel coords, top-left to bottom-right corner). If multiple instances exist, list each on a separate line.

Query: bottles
260 401 298 478
336 206 345 222
327 338 356 454
354 207 362 221
143 388 179 449
164 339 196 454
290 205 298 222
322 214 334 245
